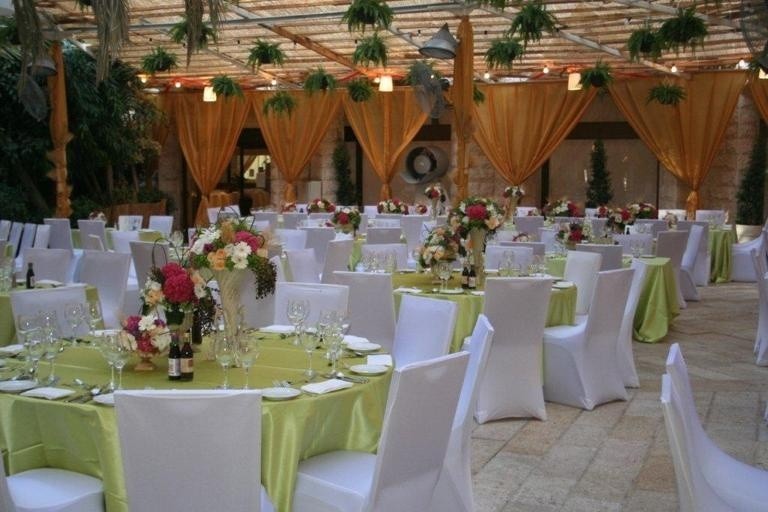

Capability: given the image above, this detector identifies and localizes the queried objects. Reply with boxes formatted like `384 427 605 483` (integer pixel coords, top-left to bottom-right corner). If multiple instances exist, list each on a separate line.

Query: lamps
26 41 59 77
417 22 461 60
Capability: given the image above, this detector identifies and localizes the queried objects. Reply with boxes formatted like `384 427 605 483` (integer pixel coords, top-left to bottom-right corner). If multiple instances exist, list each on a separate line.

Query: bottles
180 329 193 382
168 335 180 379
626 228 630 234
468 265 476 289
27 263 35 289
462 266 468 287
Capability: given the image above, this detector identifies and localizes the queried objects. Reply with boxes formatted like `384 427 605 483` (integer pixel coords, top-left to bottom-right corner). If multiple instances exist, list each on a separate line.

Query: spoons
354 350 393 355
327 368 368 385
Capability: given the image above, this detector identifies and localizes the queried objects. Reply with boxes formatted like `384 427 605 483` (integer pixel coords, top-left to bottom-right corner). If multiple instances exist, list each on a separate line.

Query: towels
0 344 25 357
302 377 353 395
472 290 486 297
393 287 424 296
36 280 61 288
19 386 76 402
260 324 296 335
341 335 367 343
366 353 392 367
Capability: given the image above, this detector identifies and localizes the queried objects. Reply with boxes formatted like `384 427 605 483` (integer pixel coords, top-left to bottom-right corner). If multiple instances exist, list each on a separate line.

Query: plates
471 290 484 295
349 364 388 377
1 380 38 395
440 287 464 295
344 342 384 353
554 280 571 289
258 387 299 400
88 328 125 336
94 392 115 405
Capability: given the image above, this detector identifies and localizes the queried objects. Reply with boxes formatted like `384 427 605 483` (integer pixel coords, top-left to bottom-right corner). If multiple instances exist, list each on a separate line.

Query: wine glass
384 253 397 272
83 300 102 344
234 338 259 388
214 337 240 388
40 334 64 386
63 302 86 346
538 255 552 276
16 311 41 360
98 332 125 388
299 323 324 378
321 326 348 380
287 299 310 346
504 250 515 269
436 260 448 290
510 262 522 276
524 255 541 275
498 261 509 276
439 263 451 290
23 330 50 382
35 310 60 359
111 337 132 387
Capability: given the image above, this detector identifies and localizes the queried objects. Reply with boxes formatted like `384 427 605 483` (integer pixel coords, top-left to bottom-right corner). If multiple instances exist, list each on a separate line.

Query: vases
135 350 157 372
208 266 250 338
140 261 221 336
431 265 445 285
613 222 625 234
162 308 198 345
469 231 488 292
565 240 582 250
431 199 439 221
508 196 519 218
117 314 173 353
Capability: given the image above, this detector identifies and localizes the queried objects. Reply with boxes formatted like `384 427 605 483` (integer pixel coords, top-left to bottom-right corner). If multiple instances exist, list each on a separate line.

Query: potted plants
338 1 394 32
578 51 614 90
645 74 686 107
735 119 767 242
352 31 389 68
507 2 568 51
742 51 768 75
142 44 178 74
628 29 661 63
347 77 375 104
244 36 289 74
304 65 335 98
657 5 708 54
482 38 524 73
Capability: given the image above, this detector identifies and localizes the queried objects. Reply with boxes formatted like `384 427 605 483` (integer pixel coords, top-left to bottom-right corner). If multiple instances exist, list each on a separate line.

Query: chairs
309 212 330 220
676 221 711 287
43 218 73 248
575 244 624 270
658 208 687 222
293 350 470 511
88 234 105 252
732 221 768 282
401 214 424 245
188 228 208 247
149 215 174 239
464 277 553 425
8 283 91 344
500 241 545 256
283 213 309 229
332 270 396 353
274 282 349 334
665 342 768 511
495 230 520 243
206 205 241 224
0 220 12 242
301 227 336 273
584 208 596 218
359 213 369 233
296 203 307 211
430 313 496 511
614 234 653 254
74 251 131 329
564 249 602 325
118 215 143 231
129 241 170 289
256 213 278 228
284 248 319 281
366 227 403 245
657 230 689 309
617 258 649 388
392 293 457 371
635 220 669 238
542 268 636 412
540 229 558 252
696 209 726 224
484 244 534 272
8 222 23 258
15 222 37 265
320 238 354 283
114 389 277 512
514 215 544 237
77 219 106 249
111 230 138 253
681 224 704 301
364 206 378 218
361 243 407 269
750 248 768 365
660 374 737 512
0 453 104 512
274 229 307 251
252 219 269 232
515 207 536 217
22 248 70 283
34 225 51 249
239 255 286 328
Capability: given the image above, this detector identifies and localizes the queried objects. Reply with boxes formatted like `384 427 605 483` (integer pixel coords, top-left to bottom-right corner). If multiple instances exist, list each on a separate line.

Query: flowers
187 214 283 299
512 231 532 243
447 195 505 240
554 221 590 243
376 198 410 215
606 207 633 229
415 203 428 213
542 197 579 217
593 206 609 217
424 182 447 203
502 185 525 199
324 207 361 234
306 199 337 215
628 201 658 219
419 226 467 268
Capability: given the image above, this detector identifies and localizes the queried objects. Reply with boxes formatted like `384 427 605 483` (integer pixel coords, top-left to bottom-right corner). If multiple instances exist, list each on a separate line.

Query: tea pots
361 250 380 272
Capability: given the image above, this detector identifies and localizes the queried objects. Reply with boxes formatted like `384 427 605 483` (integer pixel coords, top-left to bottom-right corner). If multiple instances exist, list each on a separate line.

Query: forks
269 378 303 402
